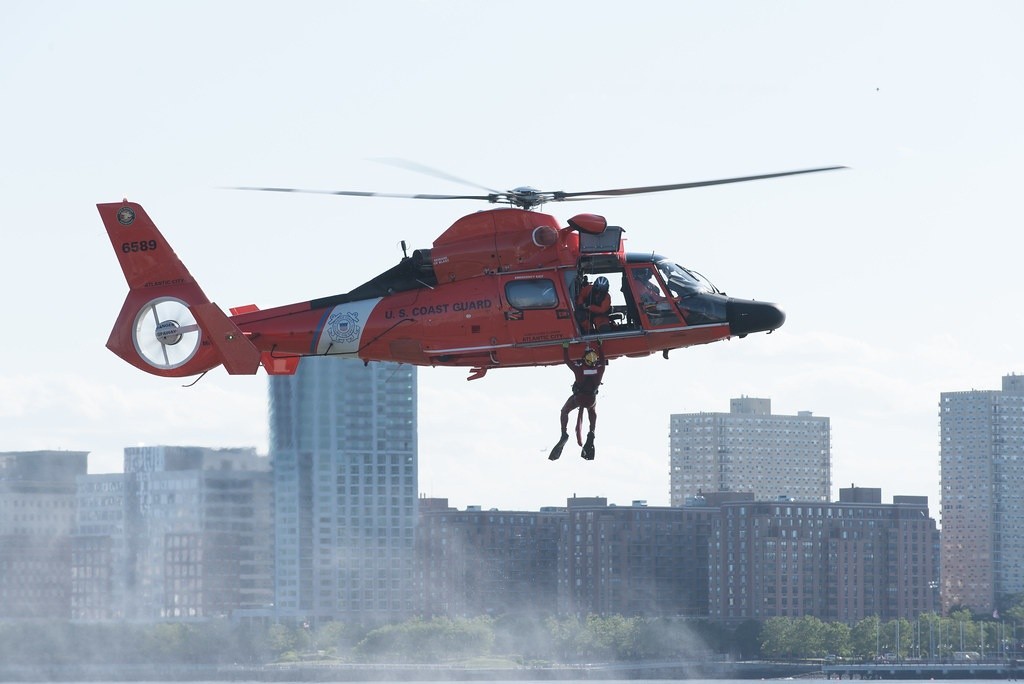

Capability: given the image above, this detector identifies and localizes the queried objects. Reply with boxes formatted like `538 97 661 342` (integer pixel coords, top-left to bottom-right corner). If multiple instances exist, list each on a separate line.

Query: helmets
594 277 609 291
639 268 652 279
584 349 597 366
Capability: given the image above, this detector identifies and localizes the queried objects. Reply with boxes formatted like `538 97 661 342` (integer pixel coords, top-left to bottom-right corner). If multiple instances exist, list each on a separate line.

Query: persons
576 276 612 334
548 337 605 460
631 267 700 324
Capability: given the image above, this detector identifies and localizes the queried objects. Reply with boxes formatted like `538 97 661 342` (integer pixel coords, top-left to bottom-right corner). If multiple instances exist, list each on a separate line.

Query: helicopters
96 154 848 381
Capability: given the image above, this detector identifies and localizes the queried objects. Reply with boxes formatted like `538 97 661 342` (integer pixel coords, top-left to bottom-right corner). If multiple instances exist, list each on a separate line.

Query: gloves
596 337 602 346
562 340 569 348
